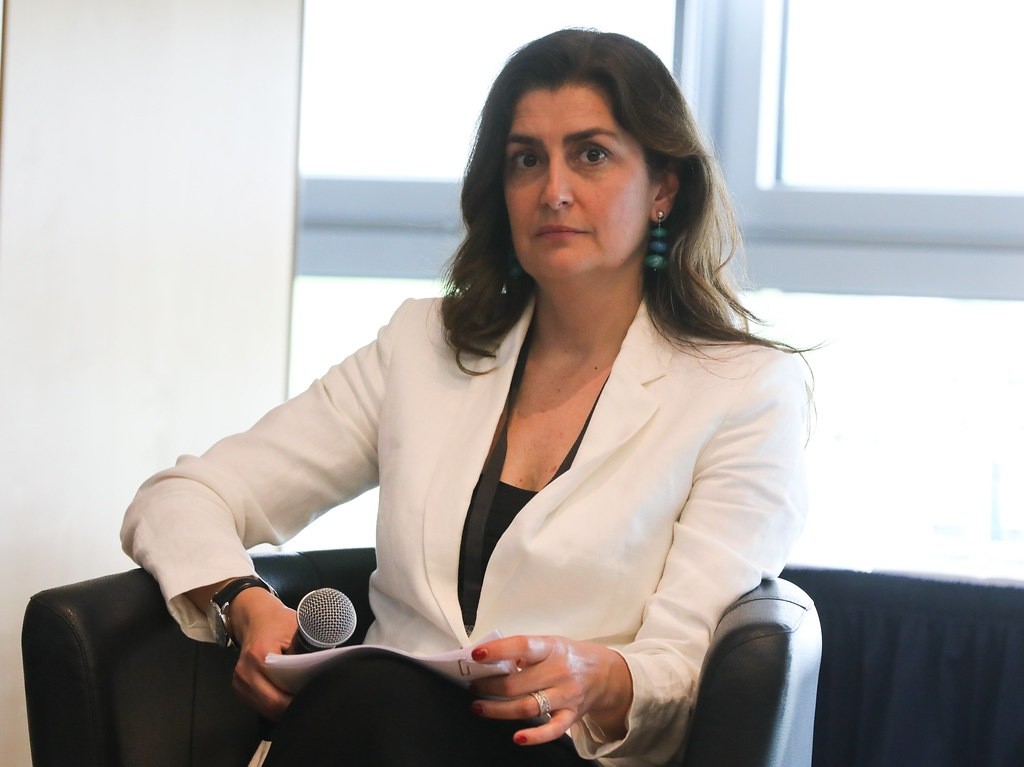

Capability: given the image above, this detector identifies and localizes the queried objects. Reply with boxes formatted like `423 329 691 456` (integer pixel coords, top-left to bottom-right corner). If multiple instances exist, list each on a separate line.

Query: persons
119 31 804 767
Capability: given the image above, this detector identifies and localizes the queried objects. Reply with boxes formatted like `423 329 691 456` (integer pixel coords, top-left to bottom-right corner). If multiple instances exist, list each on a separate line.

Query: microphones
257 588 359 742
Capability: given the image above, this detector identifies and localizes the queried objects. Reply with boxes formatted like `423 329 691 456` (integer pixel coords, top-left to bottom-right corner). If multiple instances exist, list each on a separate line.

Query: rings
530 690 551 717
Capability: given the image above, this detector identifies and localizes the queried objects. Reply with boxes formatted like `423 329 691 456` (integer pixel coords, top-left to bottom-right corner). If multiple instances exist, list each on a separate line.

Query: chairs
21 548 821 767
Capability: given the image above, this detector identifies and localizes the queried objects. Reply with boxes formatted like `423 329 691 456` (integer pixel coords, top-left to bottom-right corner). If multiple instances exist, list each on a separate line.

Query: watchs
206 578 274 648
